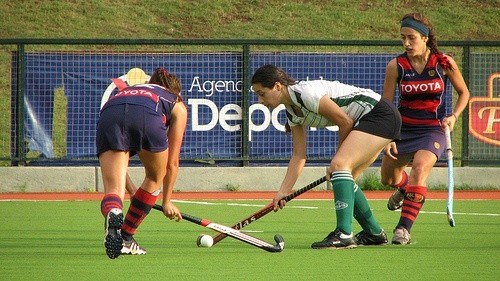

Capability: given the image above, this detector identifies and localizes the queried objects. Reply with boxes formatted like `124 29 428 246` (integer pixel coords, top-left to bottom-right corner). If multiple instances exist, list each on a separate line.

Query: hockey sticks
151 203 285 253
444 124 457 229
196 173 332 248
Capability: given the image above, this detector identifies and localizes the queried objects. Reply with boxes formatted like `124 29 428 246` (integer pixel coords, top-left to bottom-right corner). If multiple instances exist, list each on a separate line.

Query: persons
380 12 470 245
250 65 401 249
95 68 188 259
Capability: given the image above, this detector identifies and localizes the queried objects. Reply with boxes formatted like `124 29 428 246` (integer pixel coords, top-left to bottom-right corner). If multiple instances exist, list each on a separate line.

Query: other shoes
387 188 405 210
391 226 411 244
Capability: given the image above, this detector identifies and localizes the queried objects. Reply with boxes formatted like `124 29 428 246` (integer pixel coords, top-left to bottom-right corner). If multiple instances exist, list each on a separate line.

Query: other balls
200 234 215 248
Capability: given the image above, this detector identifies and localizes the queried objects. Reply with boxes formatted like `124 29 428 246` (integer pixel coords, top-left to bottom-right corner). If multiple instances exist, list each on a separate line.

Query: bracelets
450 113 458 124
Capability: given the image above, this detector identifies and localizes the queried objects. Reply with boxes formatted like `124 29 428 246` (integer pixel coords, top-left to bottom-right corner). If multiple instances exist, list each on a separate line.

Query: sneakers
312 228 357 249
119 234 147 255
103 207 125 259
354 228 389 246
